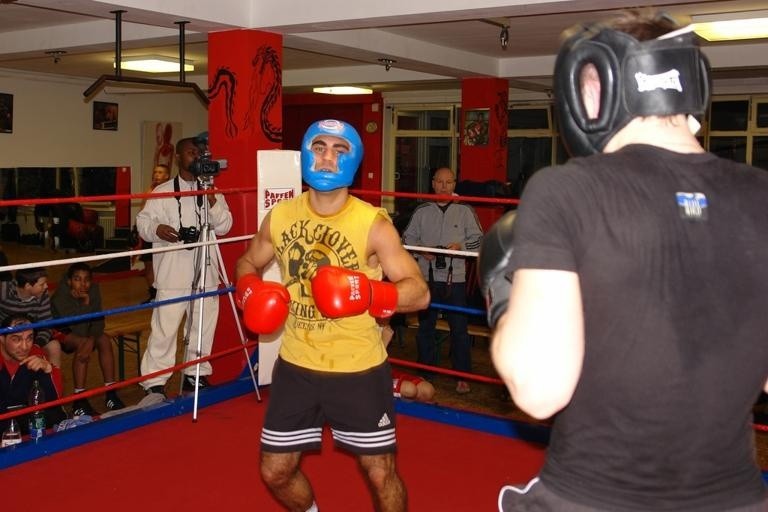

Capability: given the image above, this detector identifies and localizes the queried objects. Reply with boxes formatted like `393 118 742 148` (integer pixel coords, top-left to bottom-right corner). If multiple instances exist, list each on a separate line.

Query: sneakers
54 416 94 432
105 397 125 411
143 385 167 403
71 398 100 417
182 375 210 392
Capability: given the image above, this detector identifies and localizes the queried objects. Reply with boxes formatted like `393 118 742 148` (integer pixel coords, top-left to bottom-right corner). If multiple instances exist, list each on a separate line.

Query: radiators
98 216 116 249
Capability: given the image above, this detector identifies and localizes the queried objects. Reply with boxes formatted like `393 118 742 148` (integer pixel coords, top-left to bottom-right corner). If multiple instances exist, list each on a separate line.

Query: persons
135 137 240 402
234 117 432 512
475 2 768 512
0 266 63 399
49 263 124 417
0 313 68 441
402 165 485 393
153 123 175 178
153 123 163 167
140 164 171 305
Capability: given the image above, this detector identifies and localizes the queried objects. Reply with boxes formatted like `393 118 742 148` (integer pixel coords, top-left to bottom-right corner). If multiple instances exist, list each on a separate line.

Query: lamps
691 10 768 42
313 86 373 95
113 55 196 73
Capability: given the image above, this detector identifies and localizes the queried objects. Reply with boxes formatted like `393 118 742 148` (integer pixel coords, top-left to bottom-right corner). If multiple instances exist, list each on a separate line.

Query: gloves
234 272 290 334
310 265 398 318
477 209 518 329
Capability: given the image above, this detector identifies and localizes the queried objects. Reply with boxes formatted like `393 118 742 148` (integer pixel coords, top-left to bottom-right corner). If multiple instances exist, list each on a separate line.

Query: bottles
2 417 22 448
27 379 46 439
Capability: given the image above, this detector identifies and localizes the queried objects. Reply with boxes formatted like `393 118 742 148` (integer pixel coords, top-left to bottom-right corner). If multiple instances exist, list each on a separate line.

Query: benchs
46 275 153 381
408 316 489 337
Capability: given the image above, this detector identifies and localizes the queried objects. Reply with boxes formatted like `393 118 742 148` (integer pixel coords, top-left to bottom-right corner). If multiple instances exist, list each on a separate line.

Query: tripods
179 184 262 423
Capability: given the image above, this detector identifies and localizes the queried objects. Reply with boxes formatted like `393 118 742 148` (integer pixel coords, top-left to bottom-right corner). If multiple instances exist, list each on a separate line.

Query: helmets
554 12 716 156
300 120 363 191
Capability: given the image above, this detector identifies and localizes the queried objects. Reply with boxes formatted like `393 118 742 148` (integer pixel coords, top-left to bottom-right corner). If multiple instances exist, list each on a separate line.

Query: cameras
177 226 200 250
430 245 448 269
189 151 228 175
